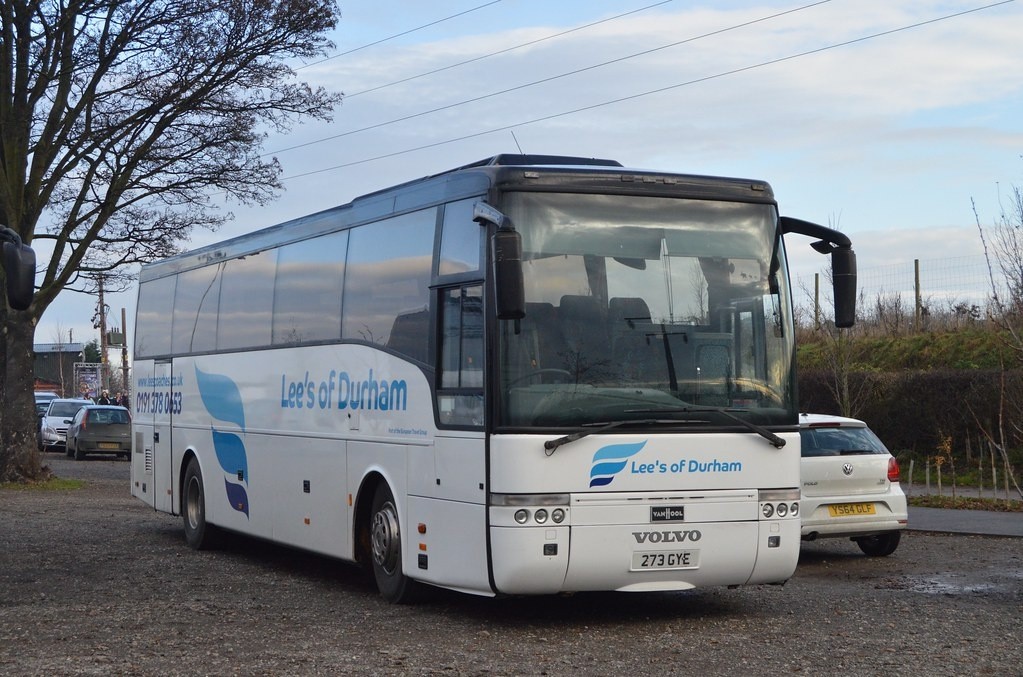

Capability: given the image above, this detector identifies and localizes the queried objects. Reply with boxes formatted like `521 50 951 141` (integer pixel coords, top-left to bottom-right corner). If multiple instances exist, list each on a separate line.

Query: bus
128 152 858 605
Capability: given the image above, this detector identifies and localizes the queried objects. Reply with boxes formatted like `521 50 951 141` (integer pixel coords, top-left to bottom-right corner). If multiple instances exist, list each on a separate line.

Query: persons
84 394 97 421
113 392 126 406
97 392 110 415
122 389 128 405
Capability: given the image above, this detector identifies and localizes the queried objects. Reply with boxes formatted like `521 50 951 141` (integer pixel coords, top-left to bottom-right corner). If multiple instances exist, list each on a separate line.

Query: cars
64 405 134 460
799 411 908 557
34 391 59 421
38 399 98 450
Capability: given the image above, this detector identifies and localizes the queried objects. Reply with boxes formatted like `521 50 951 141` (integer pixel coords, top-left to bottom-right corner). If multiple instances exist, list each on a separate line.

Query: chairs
558 295 611 368
609 297 660 363
